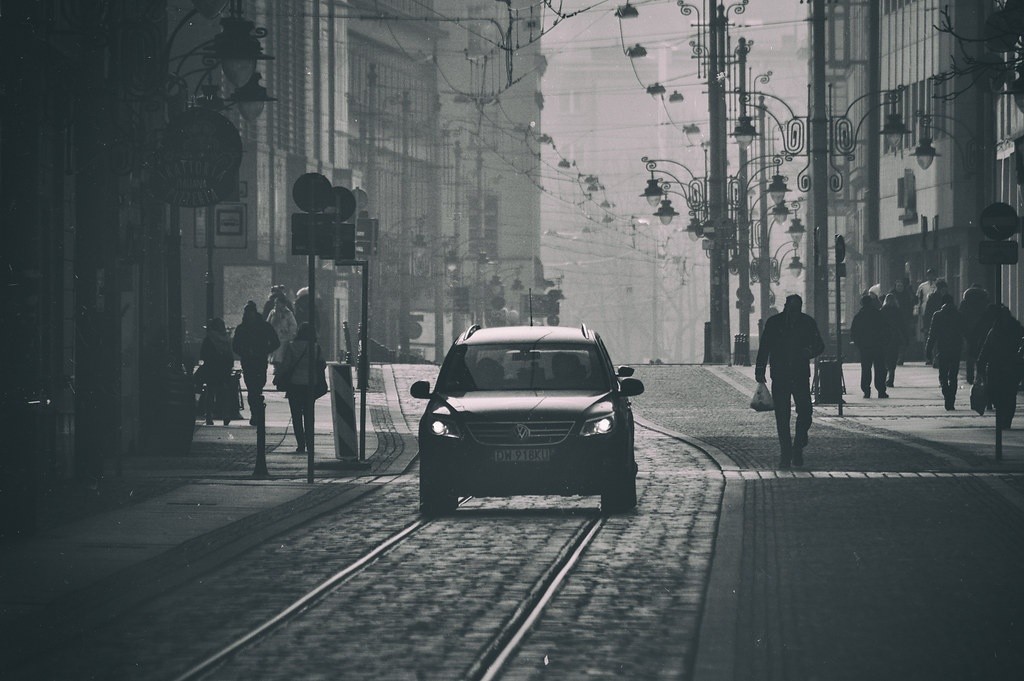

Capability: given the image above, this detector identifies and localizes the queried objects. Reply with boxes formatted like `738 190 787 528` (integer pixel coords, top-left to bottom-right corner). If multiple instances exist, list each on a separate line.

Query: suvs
409 321 646 514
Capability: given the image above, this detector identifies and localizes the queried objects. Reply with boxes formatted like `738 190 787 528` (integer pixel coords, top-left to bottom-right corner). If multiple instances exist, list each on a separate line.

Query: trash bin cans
704 322 711 362
819 360 842 405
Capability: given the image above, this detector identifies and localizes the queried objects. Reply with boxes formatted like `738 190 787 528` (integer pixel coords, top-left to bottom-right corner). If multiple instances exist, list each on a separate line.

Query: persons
755 294 824 469
850 267 1024 431
282 322 322 453
233 302 280 425
199 316 234 426
262 284 321 392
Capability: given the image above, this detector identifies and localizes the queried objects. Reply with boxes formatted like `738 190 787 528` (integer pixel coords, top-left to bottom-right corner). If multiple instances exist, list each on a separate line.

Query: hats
868 283 881 296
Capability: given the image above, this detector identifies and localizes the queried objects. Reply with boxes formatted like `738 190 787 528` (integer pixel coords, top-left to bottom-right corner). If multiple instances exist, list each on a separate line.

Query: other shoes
779 455 790 468
792 446 804 467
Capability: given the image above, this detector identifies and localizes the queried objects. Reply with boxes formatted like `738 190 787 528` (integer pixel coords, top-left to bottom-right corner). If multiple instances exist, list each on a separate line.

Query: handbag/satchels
316 341 328 399
970 378 987 415
751 382 772 412
273 365 294 386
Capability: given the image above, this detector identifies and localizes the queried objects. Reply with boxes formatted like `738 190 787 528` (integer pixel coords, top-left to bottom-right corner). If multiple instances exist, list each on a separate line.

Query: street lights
349 63 562 365
730 0 916 405
145 0 279 455
614 0 809 366
186 68 280 331
